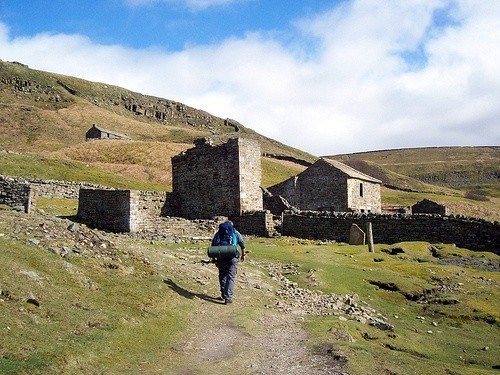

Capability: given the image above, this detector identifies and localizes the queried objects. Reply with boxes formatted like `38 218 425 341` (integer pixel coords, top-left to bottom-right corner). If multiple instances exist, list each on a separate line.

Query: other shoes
221 294 234 306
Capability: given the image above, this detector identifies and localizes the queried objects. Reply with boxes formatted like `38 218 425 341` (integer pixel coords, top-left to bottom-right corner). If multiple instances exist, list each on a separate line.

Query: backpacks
215 221 235 260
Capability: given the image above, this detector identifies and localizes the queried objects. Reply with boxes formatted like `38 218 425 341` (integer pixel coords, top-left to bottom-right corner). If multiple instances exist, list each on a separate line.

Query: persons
209 219 247 306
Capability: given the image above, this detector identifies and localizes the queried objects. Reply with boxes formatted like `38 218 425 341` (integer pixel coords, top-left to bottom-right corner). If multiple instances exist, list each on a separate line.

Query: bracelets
242 254 246 256
